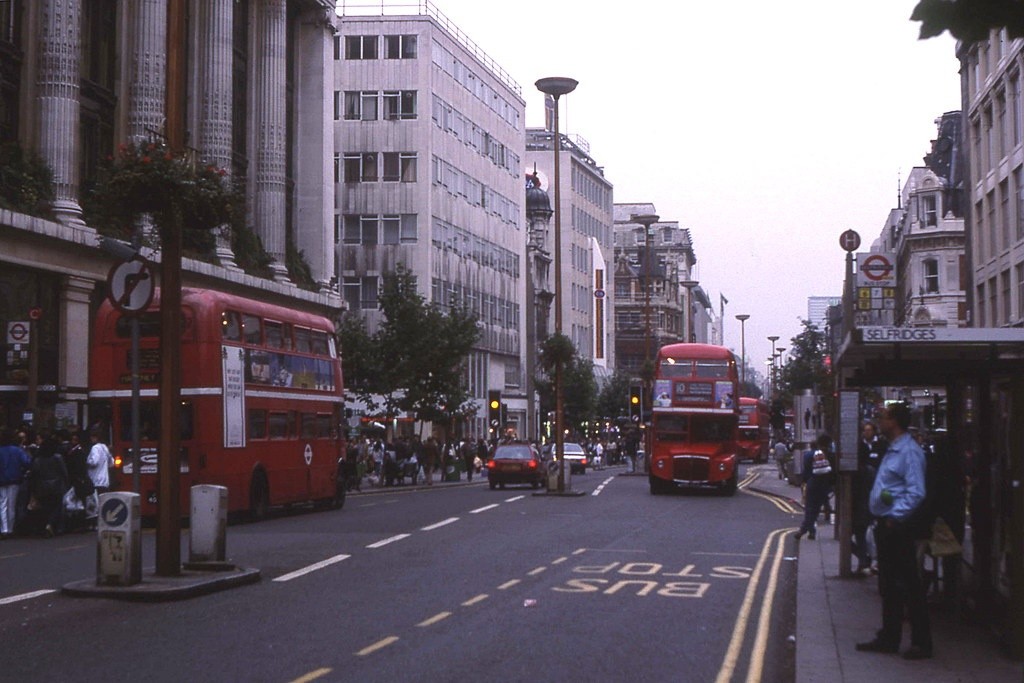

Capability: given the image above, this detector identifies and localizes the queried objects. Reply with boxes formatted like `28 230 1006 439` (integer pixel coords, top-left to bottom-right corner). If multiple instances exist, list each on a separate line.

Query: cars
488 446 544 489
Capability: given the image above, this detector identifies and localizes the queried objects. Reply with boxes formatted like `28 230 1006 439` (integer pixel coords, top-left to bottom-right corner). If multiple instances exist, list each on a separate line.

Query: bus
85 287 348 522
738 397 770 462
645 343 741 495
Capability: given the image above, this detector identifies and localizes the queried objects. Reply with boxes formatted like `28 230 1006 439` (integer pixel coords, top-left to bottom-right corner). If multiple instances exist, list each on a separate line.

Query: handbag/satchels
819 472 838 493
944 556 983 603
928 517 963 557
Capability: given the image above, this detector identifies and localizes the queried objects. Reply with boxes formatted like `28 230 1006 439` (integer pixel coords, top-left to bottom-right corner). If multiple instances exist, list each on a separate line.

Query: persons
717 392 733 409
815 445 836 522
564 417 643 474
915 432 966 597
775 439 791 479
804 408 817 430
851 422 890 576
855 402 933 660
656 391 671 407
794 442 827 540
0 422 116 539
343 429 556 493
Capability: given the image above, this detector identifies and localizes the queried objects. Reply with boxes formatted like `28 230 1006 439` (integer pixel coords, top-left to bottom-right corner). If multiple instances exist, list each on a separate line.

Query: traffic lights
489 389 500 426
629 386 643 424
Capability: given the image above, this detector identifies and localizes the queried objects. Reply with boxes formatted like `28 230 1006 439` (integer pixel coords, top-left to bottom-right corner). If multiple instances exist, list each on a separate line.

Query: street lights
679 281 698 343
634 214 660 471
768 336 786 397
535 78 579 488
736 314 751 394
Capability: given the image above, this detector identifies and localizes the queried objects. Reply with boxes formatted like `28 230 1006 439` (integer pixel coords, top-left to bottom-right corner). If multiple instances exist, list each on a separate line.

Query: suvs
550 443 587 473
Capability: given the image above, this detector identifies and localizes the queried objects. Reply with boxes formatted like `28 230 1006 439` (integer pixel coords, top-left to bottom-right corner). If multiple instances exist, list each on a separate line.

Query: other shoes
902 645 933 659
808 533 815 539
794 533 801 540
856 638 900 652
